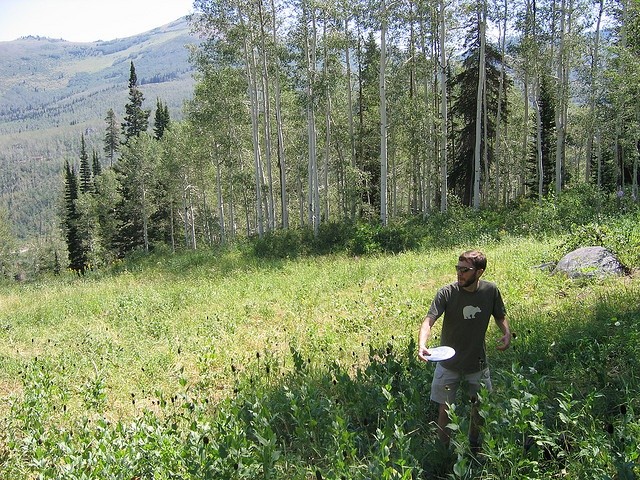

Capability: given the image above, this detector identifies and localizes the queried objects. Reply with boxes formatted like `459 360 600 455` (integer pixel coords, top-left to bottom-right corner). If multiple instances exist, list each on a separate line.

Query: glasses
455 265 476 273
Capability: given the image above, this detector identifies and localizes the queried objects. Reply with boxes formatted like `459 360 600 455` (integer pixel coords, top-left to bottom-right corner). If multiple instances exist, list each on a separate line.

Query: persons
417 248 512 459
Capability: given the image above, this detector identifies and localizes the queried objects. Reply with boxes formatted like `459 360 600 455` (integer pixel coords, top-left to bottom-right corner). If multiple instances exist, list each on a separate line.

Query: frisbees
424 347 455 362
